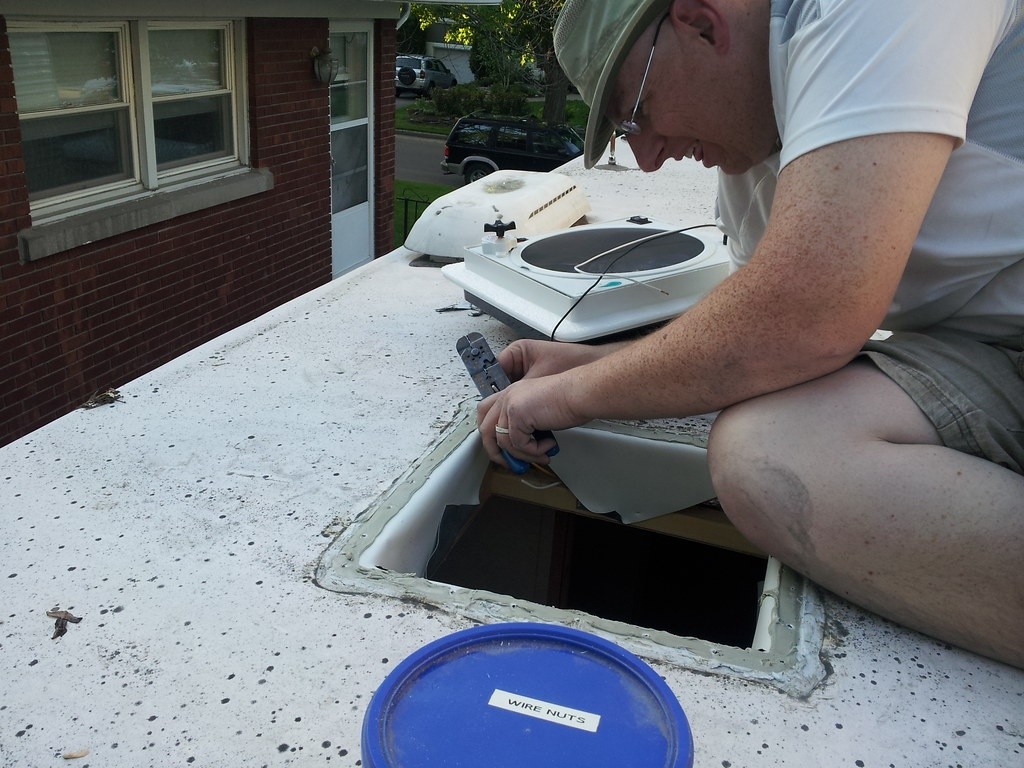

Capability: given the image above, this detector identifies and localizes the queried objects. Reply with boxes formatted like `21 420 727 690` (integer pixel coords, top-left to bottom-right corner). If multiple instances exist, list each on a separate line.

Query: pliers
456 332 561 472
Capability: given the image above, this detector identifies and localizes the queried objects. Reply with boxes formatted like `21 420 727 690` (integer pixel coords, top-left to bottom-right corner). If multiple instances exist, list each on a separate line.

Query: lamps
311 45 340 85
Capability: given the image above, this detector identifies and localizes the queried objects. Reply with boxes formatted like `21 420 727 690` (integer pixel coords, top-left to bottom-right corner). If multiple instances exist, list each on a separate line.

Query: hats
552 0 652 170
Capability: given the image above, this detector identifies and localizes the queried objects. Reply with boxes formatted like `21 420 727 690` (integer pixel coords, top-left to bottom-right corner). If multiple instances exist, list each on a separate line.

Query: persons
475 1 1024 668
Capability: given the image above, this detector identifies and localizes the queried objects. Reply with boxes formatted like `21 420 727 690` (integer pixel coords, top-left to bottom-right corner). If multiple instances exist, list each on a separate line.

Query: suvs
440 109 585 186
394 52 458 99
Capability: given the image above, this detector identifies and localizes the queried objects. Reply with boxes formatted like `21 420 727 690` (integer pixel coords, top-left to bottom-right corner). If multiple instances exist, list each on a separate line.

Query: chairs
426 61 435 71
511 139 527 152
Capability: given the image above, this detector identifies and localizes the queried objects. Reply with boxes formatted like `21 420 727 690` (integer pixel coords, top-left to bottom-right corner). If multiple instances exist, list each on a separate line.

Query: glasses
619 8 672 136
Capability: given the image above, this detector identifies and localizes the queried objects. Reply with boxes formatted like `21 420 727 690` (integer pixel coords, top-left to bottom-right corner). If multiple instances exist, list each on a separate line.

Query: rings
494 426 510 435
